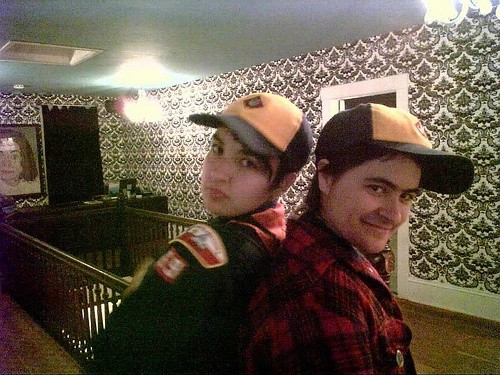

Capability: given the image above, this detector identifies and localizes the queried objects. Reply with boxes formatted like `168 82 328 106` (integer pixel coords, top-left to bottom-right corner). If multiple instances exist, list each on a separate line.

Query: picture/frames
0 124 47 199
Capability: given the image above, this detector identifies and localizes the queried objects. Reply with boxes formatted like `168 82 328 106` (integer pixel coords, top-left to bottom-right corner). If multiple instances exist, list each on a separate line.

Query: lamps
421 0 500 26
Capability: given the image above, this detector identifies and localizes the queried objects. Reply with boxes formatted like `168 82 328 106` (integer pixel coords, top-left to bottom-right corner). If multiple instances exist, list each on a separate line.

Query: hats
190 91 314 173
316 102 474 194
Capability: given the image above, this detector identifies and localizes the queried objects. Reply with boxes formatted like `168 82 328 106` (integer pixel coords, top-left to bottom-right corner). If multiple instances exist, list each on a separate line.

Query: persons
91 92 312 375
243 102 475 375
0 127 39 193
